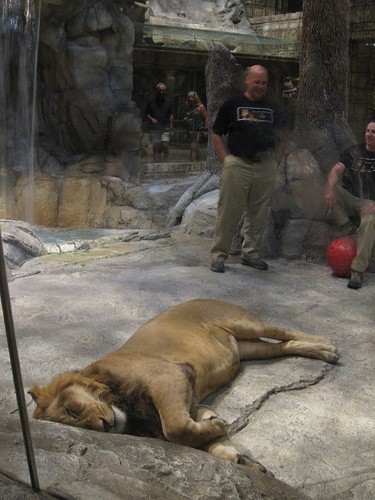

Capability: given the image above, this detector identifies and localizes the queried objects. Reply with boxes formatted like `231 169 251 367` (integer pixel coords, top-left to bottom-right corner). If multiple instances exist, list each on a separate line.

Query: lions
27 298 340 477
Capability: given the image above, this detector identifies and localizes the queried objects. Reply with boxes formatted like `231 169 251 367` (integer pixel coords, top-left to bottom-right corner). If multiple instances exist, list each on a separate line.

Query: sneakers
240 257 268 270
348 269 363 288
342 220 357 235
209 261 224 271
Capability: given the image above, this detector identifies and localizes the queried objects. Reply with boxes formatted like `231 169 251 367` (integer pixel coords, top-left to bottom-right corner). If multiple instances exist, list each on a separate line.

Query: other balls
326 237 358 278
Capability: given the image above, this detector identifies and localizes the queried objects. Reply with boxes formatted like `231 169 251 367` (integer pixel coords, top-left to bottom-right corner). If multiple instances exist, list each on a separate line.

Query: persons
211 65 285 273
324 122 375 289
184 91 207 161
145 82 171 162
